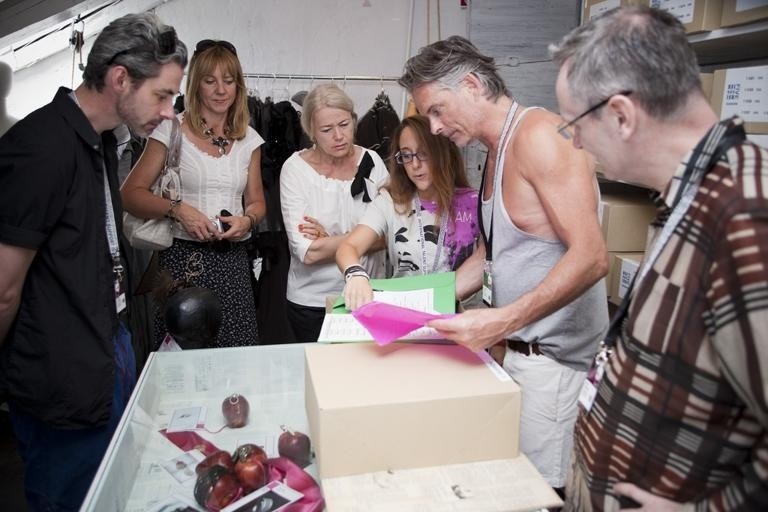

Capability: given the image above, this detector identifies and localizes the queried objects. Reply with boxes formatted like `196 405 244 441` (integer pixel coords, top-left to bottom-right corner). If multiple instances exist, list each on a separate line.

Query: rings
317 230 321 238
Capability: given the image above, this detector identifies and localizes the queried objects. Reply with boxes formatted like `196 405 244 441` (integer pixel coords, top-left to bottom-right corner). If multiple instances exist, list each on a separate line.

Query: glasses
394 150 433 165
106 30 177 67
551 89 633 140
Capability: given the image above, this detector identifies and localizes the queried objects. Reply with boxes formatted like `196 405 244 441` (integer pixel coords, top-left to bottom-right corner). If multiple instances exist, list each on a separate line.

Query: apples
222 396 249 429
279 432 310 467
195 451 232 475
232 443 268 491
195 472 237 511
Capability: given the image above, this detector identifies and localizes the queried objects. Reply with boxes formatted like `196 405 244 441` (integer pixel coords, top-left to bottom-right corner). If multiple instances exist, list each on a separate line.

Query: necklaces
197 115 232 154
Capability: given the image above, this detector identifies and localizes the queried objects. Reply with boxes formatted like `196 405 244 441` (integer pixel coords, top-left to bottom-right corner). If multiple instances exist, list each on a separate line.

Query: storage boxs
304 341 522 479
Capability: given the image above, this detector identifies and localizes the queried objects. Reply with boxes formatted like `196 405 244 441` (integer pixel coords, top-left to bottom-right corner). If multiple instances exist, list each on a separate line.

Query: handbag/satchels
122 165 181 252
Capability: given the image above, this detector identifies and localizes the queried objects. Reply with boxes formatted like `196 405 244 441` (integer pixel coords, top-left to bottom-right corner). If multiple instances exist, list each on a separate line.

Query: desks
78 343 564 512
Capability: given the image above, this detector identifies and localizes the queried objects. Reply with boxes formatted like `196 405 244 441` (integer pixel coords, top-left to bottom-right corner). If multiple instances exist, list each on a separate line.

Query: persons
398 36 610 489
120 39 267 349
551 6 767 510
276 83 390 341
0 14 186 512
335 115 481 360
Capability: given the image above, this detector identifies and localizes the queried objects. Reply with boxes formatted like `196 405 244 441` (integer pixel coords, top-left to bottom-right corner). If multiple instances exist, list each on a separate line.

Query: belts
506 339 544 356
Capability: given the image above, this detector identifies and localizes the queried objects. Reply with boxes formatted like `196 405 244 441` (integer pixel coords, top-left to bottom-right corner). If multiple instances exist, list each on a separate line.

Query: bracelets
165 198 181 232
341 266 374 279
244 212 255 229
